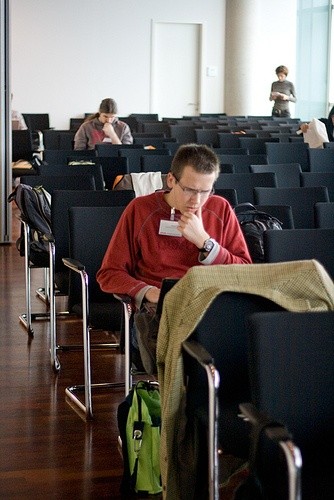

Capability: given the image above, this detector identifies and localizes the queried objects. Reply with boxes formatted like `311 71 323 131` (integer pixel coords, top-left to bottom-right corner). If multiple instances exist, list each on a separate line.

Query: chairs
11 113 334 500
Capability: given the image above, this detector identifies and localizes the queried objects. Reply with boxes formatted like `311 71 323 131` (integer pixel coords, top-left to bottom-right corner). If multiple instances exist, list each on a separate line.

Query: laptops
142 278 181 315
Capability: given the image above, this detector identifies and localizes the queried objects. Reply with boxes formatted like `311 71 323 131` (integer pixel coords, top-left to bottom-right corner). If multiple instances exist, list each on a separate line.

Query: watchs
199 239 215 253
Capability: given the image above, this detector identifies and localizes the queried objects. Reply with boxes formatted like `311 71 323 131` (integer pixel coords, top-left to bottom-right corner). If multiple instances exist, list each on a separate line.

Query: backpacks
117 379 164 500
7 182 54 264
232 202 284 263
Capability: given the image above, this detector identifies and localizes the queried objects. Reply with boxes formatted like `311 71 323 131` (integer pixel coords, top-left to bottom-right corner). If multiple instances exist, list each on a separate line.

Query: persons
298 105 334 142
74 99 133 150
270 65 296 117
96 144 253 378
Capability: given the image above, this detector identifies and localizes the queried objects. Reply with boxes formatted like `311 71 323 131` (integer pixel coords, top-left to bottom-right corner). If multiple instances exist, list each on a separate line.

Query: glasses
171 173 215 198
